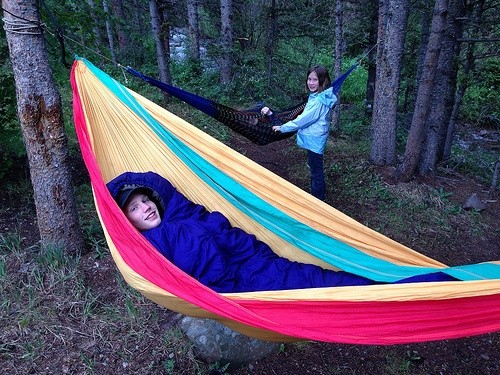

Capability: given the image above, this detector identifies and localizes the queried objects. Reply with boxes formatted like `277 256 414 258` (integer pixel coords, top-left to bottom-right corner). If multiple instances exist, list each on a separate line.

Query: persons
271 64 338 202
107 170 463 290
237 103 285 128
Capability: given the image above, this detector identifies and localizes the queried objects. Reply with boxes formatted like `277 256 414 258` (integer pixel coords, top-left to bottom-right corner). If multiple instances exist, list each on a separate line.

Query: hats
117 186 154 209
